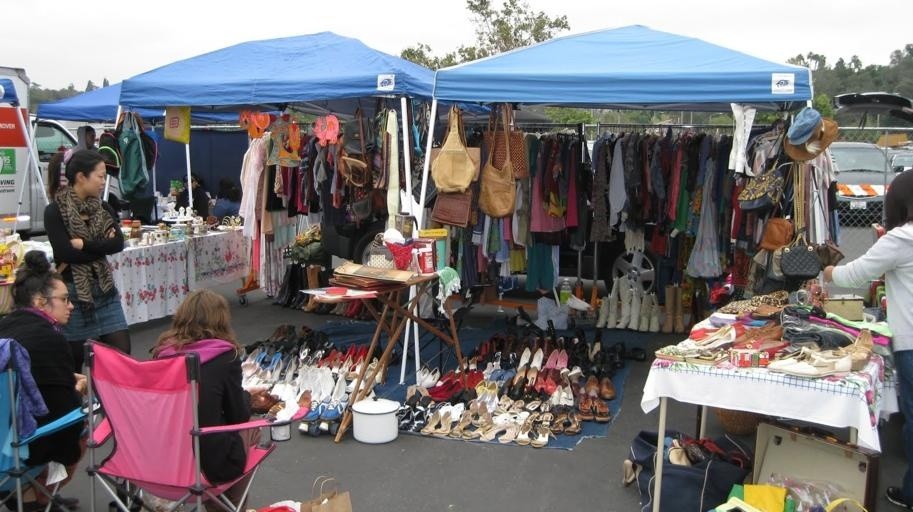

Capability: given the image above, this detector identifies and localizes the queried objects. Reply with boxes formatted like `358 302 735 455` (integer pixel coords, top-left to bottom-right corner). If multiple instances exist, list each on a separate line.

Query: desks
104 225 252 326
638 304 899 511
313 266 468 443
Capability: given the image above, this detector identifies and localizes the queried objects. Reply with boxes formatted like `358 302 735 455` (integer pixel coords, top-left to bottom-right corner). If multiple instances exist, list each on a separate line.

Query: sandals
822 340 856 361
847 329 876 376
395 319 647 449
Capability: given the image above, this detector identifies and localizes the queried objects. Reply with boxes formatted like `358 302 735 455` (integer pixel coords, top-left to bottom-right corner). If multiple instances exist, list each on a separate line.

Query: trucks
0 70 123 241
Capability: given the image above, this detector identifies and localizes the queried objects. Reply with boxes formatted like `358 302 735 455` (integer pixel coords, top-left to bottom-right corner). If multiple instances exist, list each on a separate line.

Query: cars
824 136 913 225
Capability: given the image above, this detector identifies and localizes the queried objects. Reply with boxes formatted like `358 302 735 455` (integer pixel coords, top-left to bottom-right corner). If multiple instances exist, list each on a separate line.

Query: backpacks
96 111 162 207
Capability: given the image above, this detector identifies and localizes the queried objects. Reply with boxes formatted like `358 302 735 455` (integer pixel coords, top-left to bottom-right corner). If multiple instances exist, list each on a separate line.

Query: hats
785 107 822 147
780 117 839 164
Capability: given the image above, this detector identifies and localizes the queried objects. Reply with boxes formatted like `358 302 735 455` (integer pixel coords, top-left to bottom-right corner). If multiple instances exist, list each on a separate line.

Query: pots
349 395 405 444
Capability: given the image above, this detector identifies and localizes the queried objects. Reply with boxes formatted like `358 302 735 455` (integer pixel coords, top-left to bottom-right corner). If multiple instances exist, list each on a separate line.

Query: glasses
39 290 71 303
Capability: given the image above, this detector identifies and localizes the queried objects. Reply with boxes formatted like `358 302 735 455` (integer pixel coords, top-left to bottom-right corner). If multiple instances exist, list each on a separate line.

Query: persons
215 175 240 220
820 167 911 511
150 288 265 512
63 124 97 164
0 249 89 512
42 148 130 373
174 172 207 218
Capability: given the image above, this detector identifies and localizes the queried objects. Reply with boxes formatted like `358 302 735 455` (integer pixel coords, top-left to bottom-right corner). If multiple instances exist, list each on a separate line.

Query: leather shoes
768 342 834 372
788 356 854 379
228 322 383 438
19 496 80 511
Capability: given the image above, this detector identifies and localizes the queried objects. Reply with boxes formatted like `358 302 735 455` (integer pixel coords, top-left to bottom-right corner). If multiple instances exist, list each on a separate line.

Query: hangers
533 111 792 154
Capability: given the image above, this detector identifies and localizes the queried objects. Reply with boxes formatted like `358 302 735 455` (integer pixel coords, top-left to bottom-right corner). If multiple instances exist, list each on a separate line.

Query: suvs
339 104 682 305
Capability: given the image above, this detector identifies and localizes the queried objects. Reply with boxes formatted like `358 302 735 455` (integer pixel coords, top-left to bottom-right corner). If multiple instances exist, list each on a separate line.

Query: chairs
80 337 278 512
0 335 102 511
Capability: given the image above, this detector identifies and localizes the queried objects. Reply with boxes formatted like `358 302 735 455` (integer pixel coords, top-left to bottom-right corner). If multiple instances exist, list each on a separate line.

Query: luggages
751 421 881 512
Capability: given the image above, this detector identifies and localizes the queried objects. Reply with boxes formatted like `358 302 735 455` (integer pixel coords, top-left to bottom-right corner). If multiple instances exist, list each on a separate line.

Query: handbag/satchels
628 427 755 512
325 102 527 239
735 156 847 283
299 474 354 512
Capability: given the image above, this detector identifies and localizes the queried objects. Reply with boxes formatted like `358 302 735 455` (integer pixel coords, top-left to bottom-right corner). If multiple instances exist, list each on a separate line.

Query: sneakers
884 485 913 512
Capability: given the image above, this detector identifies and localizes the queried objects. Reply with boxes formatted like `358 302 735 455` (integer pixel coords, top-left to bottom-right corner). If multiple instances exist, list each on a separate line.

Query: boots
274 261 382 317
596 276 706 335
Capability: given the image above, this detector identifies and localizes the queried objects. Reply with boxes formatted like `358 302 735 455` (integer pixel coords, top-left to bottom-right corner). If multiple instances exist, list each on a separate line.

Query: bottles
559 277 573 306
121 219 141 239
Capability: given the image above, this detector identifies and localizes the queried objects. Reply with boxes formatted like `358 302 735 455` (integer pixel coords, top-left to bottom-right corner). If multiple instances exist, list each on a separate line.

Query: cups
419 247 435 273
